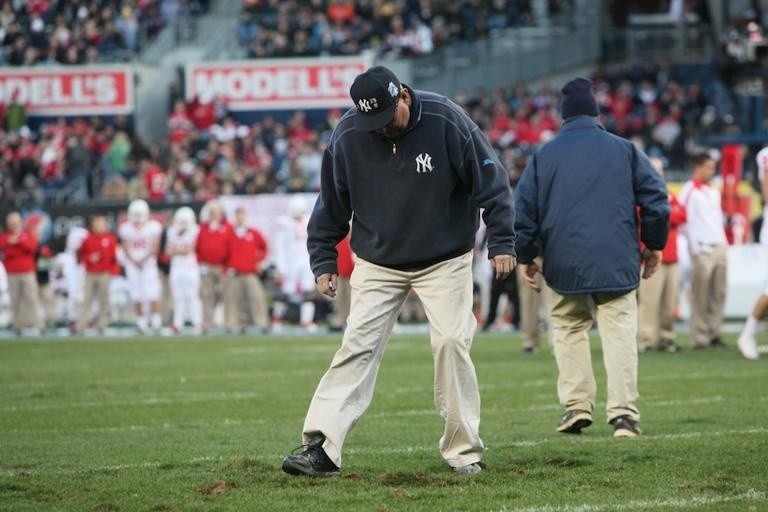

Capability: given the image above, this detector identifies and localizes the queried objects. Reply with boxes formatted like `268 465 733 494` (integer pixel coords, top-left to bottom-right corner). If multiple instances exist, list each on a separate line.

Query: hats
348 68 404 132
556 78 602 118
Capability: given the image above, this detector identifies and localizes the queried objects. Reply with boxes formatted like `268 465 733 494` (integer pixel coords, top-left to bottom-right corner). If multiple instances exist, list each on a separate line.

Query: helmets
128 198 150 221
174 206 197 228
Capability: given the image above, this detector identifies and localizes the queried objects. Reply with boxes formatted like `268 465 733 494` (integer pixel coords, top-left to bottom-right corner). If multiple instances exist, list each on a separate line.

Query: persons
280 67 516 479
513 78 671 435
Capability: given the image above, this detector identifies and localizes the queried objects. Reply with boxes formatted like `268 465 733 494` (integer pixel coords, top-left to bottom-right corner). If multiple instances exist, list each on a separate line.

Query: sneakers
734 332 759 360
612 416 643 438
454 464 485 476
641 338 681 354
693 337 732 352
280 432 340 478
555 408 593 434
